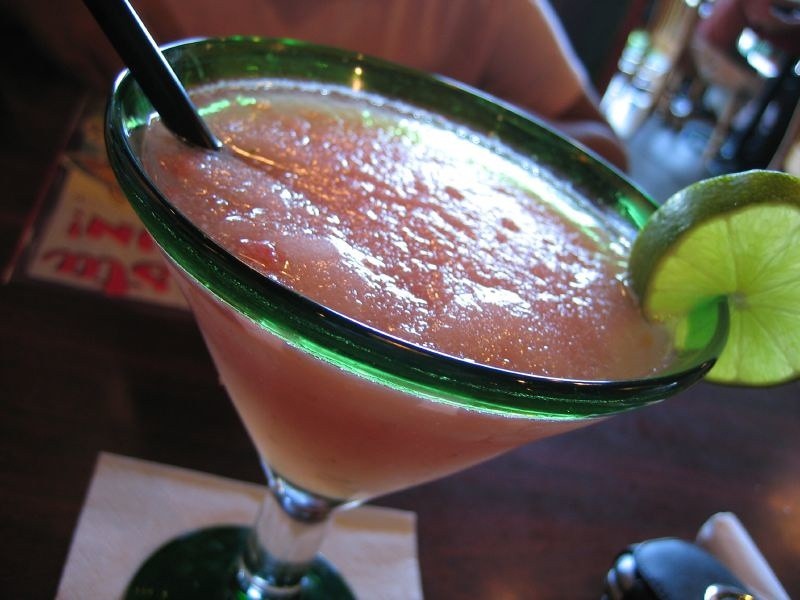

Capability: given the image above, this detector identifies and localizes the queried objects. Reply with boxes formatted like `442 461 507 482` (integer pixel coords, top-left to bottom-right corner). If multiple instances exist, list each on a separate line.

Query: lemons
627 169 799 386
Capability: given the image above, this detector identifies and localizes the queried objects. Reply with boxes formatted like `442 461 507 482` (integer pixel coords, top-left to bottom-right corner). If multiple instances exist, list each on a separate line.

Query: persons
10 0 631 176
690 0 800 163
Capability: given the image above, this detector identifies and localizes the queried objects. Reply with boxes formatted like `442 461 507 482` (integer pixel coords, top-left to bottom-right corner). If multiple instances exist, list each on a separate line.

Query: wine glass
101 34 731 599
